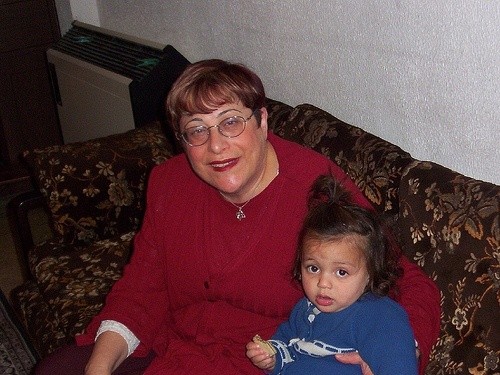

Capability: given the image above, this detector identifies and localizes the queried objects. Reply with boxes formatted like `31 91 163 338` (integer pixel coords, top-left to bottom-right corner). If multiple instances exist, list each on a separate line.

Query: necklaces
217 143 268 220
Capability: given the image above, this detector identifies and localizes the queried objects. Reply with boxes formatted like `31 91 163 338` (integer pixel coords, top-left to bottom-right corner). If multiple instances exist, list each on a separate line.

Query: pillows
15 111 184 254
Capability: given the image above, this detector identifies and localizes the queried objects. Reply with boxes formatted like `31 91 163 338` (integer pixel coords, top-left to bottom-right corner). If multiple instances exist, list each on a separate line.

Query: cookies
253 335 277 357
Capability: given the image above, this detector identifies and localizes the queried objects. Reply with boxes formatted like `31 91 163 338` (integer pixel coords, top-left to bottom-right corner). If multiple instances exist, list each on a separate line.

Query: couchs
9 98 500 375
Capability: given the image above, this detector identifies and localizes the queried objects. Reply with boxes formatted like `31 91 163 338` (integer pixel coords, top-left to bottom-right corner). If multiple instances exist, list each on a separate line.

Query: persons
246 172 420 375
29 58 440 375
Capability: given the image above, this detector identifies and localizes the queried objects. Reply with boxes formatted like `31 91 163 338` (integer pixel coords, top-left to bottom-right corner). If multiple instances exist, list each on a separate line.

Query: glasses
178 107 258 147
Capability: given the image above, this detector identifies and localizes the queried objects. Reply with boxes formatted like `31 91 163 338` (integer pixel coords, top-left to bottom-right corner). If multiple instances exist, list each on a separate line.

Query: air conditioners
46 20 169 144
